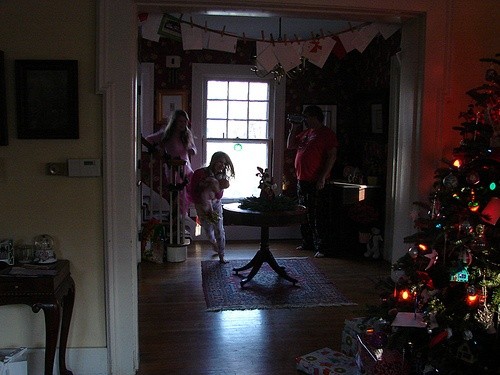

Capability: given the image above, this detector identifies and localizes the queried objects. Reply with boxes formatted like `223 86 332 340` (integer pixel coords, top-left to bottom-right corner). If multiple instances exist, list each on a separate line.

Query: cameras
287 114 305 123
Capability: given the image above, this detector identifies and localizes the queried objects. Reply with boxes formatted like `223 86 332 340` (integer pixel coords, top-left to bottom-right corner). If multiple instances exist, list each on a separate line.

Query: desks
0 260 75 375
223 202 307 287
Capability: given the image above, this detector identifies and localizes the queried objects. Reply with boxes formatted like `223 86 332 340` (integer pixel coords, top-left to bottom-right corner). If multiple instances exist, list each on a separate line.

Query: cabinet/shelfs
326 180 383 257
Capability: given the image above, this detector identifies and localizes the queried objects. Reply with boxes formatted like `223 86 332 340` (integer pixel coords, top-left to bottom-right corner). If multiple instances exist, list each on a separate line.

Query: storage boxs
296 347 358 375
342 322 358 354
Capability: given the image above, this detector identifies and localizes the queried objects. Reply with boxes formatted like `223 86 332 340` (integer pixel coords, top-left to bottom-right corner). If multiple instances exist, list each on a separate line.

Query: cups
15 234 57 264
348 172 365 185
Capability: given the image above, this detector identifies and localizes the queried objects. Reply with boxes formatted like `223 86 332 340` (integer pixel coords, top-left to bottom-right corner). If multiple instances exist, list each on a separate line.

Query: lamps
250 44 307 85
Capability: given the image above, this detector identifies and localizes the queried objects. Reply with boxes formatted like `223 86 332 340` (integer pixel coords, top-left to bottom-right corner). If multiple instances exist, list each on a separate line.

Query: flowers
256 167 277 189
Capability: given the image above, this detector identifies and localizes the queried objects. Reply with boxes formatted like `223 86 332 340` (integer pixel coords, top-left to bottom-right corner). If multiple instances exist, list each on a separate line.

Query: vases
260 189 274 201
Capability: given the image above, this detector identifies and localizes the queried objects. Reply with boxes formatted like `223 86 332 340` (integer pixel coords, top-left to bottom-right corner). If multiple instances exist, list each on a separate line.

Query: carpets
198 256 346 311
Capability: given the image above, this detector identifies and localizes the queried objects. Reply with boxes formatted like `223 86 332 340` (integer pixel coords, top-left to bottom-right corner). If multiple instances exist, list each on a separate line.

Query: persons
192 152 233 263
287 105 337 259
145 109 197 236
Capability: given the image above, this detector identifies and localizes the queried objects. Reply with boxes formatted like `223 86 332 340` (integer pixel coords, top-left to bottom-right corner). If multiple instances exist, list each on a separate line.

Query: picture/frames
15 59 79 139
157 90 188 122
